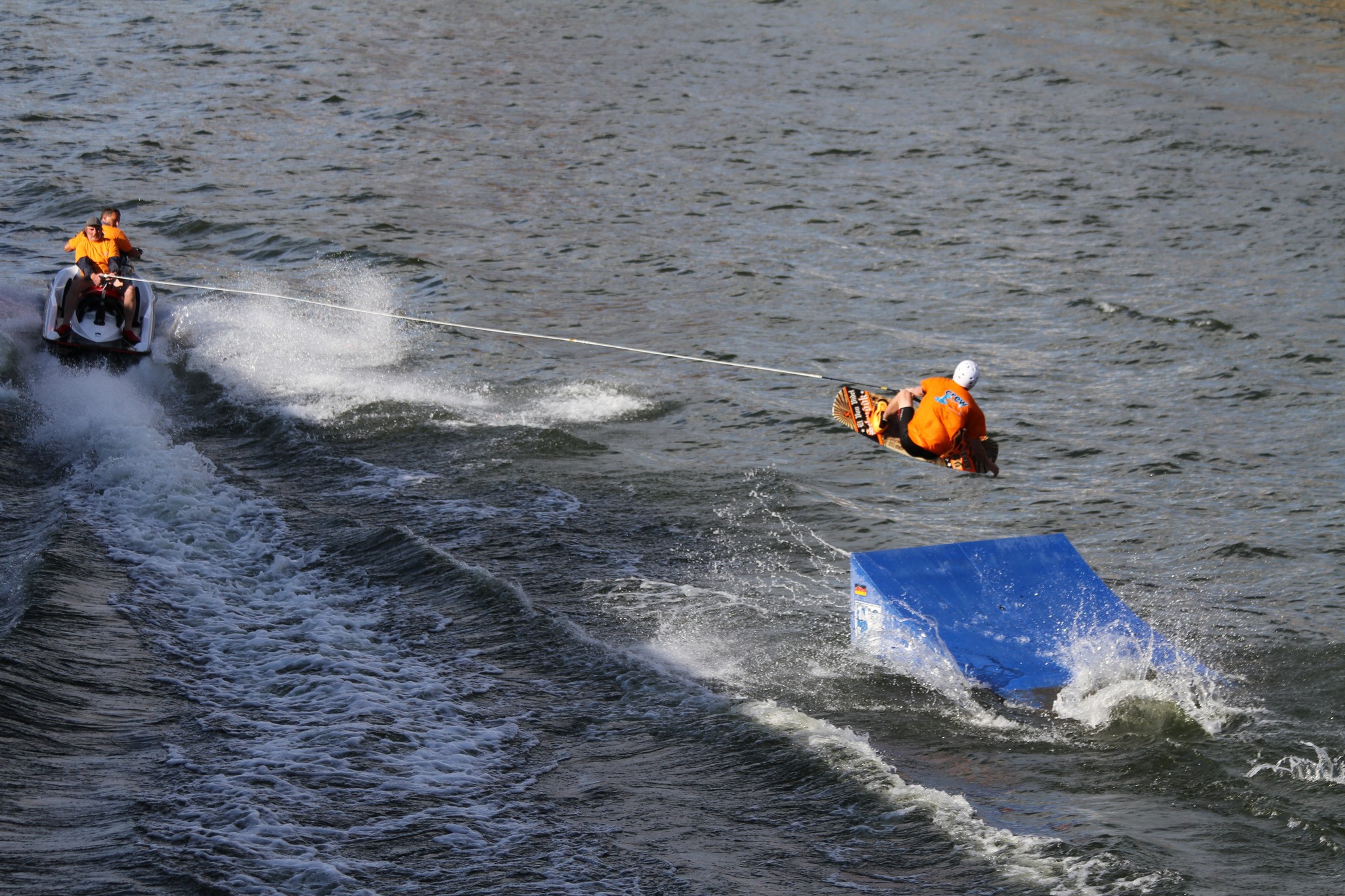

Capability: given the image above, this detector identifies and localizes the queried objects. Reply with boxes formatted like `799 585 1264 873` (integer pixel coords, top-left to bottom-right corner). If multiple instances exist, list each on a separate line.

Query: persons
65 206 141 280
871 359 987 460
47 216 147 352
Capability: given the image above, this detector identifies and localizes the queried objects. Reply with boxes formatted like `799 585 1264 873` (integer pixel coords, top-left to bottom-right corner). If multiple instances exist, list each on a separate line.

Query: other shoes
57 324 71 335
123 327 140 345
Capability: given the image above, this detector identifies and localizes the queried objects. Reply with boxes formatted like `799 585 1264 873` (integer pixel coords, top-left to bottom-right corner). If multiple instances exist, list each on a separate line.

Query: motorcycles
42 262 157 356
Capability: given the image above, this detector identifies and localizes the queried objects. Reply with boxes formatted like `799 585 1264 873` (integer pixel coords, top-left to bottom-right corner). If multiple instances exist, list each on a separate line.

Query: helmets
953 359 979 389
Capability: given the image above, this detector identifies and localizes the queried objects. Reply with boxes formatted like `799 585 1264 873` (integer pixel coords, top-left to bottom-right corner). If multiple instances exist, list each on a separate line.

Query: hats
86 216 102 227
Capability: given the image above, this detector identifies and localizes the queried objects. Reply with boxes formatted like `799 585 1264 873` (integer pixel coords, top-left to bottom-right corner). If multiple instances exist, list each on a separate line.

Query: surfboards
832 368 999 474
